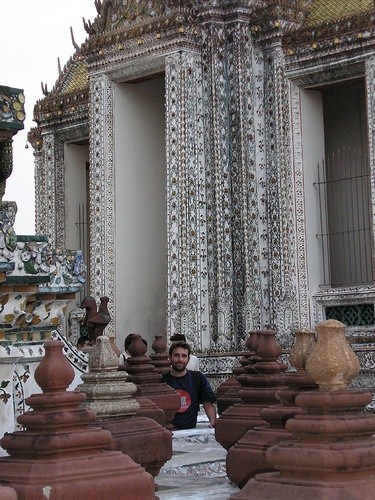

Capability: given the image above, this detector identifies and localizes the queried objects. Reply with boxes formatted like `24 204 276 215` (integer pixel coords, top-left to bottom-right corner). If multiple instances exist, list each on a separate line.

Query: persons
161 341 218 430
77 333 148 366
169 333 200 371
